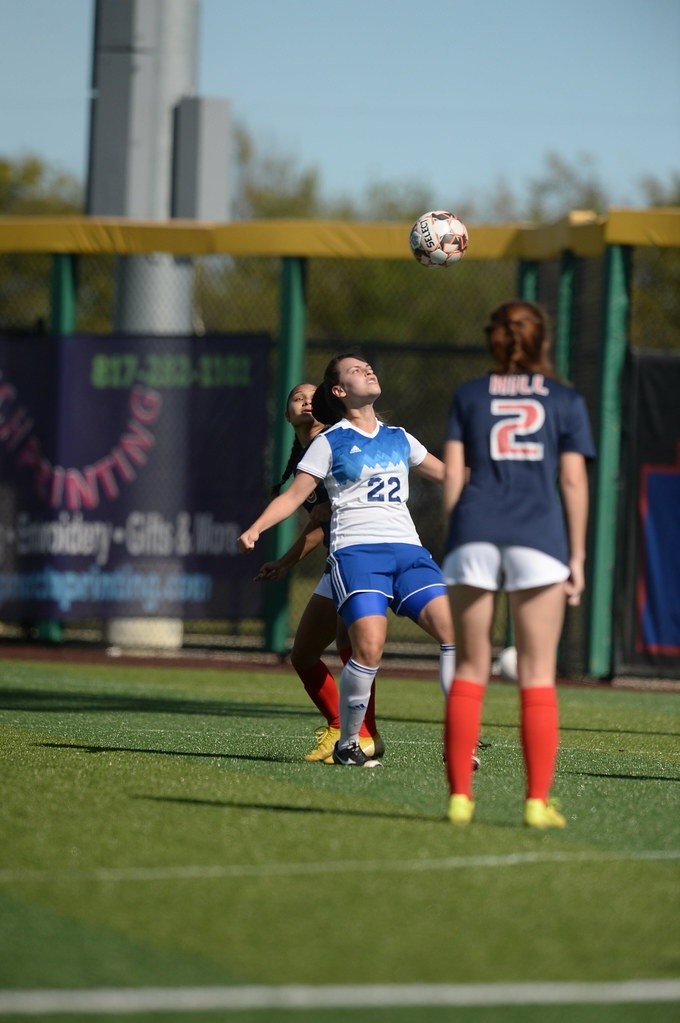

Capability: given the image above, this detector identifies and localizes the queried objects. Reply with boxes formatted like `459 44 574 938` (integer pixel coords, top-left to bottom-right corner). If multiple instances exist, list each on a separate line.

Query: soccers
410 210 469 267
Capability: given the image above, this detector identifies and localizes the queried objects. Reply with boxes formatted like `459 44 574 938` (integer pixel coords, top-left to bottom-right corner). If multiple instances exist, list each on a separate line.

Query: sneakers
523 798 567 828
471 751 480 769
304 725 340 761
324 732 385 764
332 740 381 768
446 792 476 825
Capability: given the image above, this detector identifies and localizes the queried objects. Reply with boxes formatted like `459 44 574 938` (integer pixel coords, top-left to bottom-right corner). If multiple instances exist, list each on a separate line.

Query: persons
442 301 596 834
252 382 385 764
236 353 486 773
